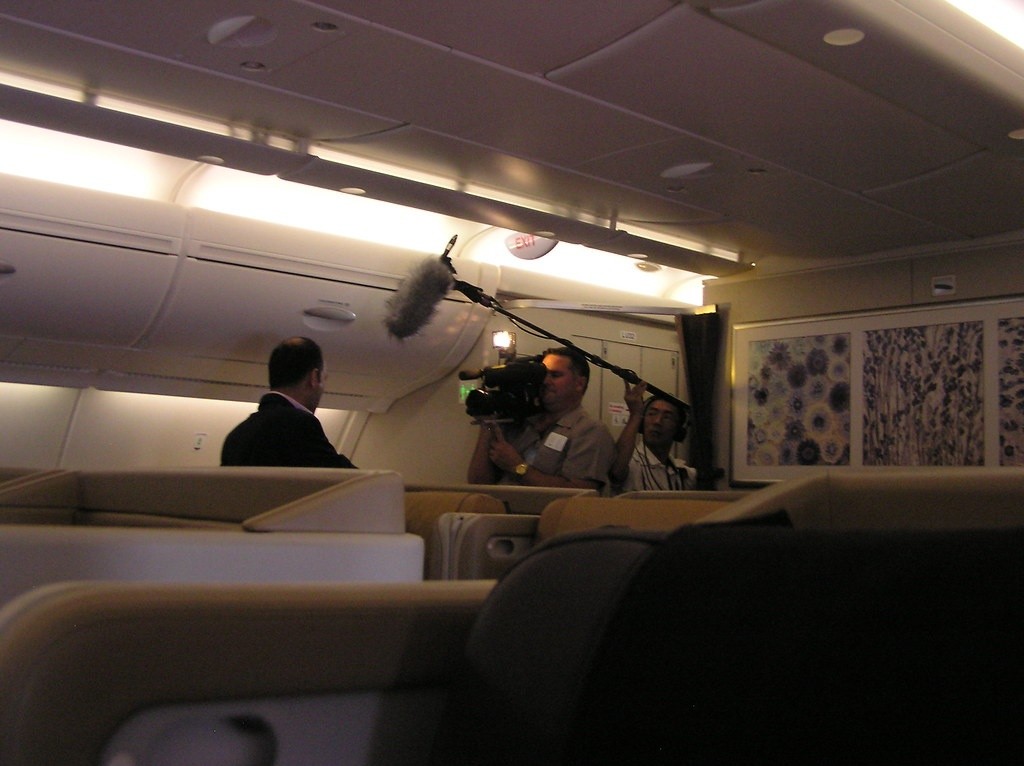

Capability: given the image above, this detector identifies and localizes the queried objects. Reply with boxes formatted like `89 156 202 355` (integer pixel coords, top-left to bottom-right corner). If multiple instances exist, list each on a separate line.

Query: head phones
638 393 689 444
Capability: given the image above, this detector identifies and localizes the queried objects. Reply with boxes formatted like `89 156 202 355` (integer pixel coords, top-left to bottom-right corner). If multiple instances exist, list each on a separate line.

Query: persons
220 336 359 469
468 347 617 496
608 380 700 494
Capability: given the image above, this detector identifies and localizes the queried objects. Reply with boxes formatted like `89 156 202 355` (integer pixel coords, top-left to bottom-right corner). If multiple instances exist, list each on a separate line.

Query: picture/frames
728 296 1024 483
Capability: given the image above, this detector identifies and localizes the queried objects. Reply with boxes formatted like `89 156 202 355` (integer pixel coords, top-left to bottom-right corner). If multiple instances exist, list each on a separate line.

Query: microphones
383 234 458 339
458 369 484 382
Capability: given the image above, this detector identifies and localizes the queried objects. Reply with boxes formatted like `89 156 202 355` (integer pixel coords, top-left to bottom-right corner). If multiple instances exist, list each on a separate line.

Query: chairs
0 464 1024 766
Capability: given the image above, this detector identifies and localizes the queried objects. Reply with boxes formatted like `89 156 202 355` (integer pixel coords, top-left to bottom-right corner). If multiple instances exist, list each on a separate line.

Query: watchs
513 464 530 480
